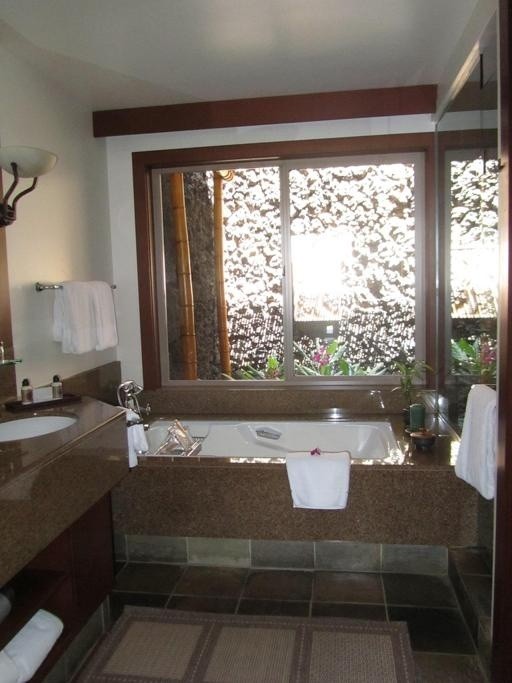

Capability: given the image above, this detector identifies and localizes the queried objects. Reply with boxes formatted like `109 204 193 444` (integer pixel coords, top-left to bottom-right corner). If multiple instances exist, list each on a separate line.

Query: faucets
1 343 5 364
117 380 151 417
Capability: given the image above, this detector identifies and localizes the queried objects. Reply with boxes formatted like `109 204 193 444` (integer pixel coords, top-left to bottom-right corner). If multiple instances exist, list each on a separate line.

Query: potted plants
391 361 434 426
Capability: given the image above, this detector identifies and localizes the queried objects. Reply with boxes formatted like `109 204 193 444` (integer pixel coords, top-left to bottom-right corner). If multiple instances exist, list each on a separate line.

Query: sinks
0 412 80 441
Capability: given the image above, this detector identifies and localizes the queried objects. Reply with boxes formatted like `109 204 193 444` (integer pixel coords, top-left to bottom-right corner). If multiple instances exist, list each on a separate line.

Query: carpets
65 606 419 682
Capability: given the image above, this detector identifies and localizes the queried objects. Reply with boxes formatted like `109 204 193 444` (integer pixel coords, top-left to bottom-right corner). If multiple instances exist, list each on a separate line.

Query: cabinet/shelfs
0 491 113 683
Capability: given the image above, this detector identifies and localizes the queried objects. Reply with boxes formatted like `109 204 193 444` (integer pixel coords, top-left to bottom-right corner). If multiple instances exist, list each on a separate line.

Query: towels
454 383 496 500
286 451 351 511
116 406 148 469
53 279 119 356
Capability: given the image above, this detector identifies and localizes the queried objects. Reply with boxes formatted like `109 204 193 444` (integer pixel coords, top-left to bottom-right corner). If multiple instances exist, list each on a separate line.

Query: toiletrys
21 379 34 405
51 375 63 400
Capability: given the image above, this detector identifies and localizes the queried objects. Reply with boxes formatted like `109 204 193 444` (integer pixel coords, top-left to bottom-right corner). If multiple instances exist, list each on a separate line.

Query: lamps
0 145 58 228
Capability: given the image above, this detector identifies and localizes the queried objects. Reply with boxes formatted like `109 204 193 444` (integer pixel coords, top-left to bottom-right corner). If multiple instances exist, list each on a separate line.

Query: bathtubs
139 419 406 468
